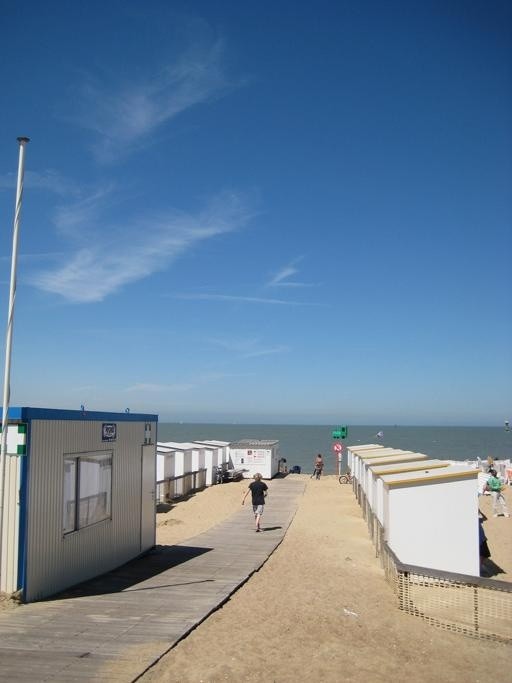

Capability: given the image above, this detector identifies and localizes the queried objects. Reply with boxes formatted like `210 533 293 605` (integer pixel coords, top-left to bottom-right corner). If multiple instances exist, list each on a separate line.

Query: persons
478 507 492 574
241 472 269 534
482 480 491 494
310 452 323 478
489 468 512 517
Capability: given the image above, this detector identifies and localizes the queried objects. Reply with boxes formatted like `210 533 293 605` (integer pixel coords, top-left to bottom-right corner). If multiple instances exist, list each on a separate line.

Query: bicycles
338 472 354 484
